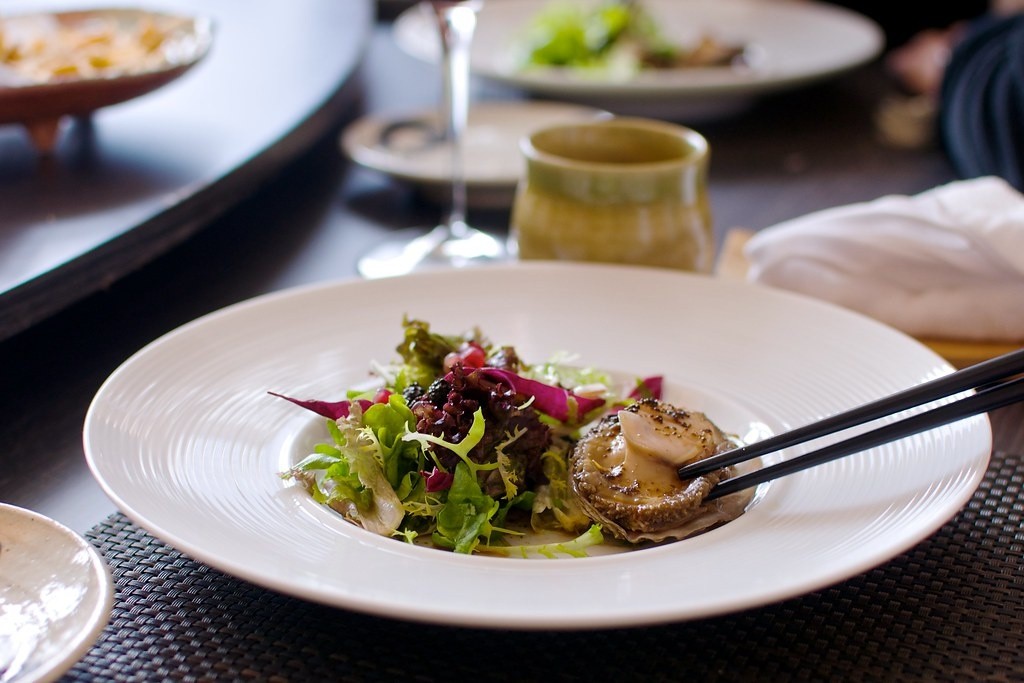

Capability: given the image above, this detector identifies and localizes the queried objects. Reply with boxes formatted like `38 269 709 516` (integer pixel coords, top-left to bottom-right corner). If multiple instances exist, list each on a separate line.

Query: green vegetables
277 314 651 556
513 0 682 73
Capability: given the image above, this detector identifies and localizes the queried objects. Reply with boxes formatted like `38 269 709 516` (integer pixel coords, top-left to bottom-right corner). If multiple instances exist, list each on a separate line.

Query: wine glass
357 0 518 277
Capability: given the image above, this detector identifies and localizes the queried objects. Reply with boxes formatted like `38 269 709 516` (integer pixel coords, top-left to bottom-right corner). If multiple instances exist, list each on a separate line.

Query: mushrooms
574 396 725 530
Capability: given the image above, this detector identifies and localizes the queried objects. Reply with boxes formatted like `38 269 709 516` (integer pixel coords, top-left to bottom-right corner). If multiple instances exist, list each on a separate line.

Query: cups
506 120 714 275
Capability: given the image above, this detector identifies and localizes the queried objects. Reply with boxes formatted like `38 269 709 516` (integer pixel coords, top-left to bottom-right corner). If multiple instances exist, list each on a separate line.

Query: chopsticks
677 348 1024 503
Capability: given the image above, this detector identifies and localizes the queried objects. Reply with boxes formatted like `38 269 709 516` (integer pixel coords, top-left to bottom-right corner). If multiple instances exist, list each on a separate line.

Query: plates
391 1 880 115
81 258 994 629
341 100 616 206
0 501 113 683
1 8 216 118
